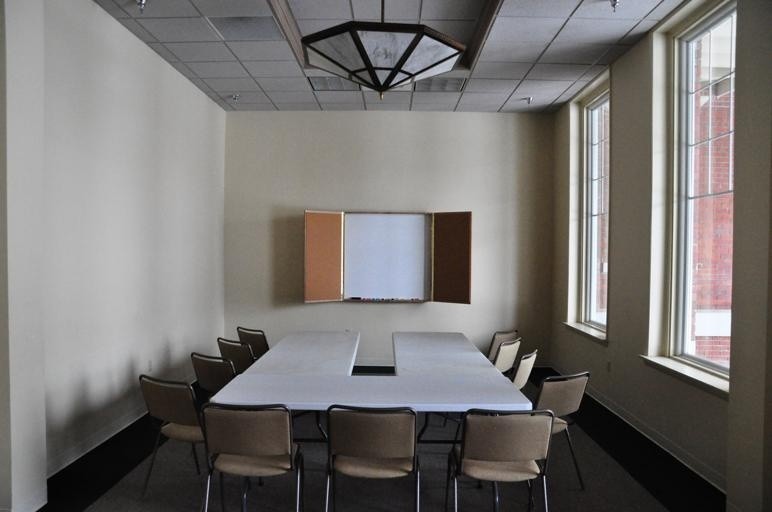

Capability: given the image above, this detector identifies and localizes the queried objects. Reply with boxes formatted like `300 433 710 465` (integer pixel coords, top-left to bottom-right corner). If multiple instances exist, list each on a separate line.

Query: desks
209 331 531 444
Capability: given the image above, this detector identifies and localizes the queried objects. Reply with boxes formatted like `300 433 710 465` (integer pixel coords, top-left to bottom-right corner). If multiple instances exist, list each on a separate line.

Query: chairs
191 352 237 392
512 349 538 389
138 374 206 495
532 372 591 490
488 330 517 360
202 402 304 512
237 327 269 359
300 21 467 101
445 408 555 512
325 404 420 512
493 338 522 373
218 338 256 374
